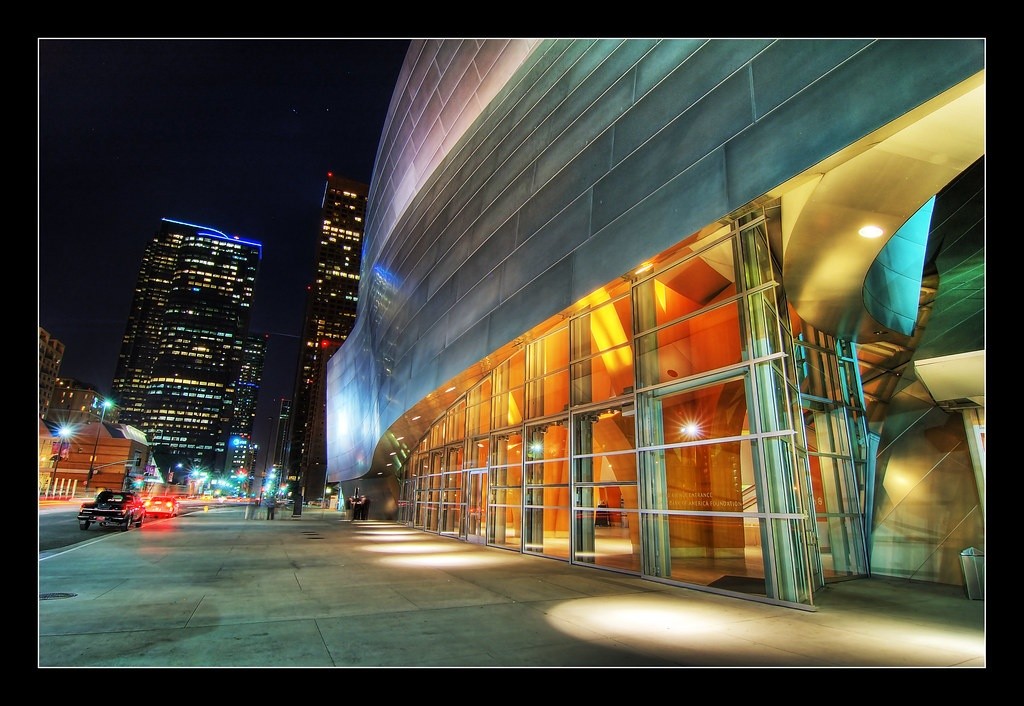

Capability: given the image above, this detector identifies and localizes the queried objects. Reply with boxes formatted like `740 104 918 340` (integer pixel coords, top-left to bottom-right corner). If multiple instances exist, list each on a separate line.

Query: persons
346 495 372 520
265 495 277 520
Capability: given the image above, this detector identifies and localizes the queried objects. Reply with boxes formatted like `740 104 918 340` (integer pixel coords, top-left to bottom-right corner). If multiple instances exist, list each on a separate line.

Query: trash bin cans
254 500 259 507
244 505 255 520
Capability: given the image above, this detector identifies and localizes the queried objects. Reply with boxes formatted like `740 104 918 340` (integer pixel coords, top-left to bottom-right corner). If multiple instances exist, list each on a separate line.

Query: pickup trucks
77 490 147 531
143 496 180 520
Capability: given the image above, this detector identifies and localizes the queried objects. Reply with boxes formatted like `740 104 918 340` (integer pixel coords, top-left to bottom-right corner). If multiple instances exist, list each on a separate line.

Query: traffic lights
136 457 141 466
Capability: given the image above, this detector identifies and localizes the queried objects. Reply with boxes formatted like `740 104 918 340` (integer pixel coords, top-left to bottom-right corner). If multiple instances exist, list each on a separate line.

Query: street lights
49 429 69 490
85 400 113 490
